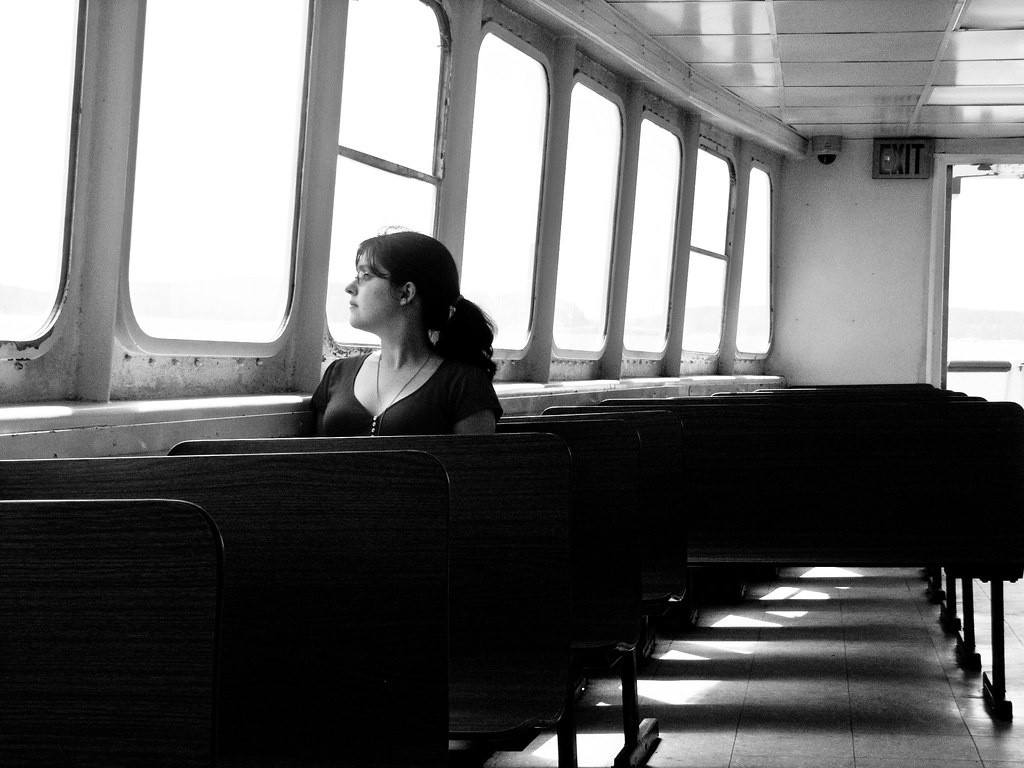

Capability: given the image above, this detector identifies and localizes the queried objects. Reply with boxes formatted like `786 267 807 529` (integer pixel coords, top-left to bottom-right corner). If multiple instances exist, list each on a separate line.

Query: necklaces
375 347 436 413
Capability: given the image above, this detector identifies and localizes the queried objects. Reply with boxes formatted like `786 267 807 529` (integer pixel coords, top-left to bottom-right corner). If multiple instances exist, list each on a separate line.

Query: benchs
1 381 1024 767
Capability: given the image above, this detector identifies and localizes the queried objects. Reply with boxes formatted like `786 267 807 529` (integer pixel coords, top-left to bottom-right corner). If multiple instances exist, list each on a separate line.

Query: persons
290 230 505 436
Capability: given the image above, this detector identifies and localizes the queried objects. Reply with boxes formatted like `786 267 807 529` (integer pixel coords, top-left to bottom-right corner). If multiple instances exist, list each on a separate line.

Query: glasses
348 268 392 286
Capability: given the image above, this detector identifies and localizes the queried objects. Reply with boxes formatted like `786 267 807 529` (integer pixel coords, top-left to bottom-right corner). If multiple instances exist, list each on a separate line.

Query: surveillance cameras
811 135 843 165
